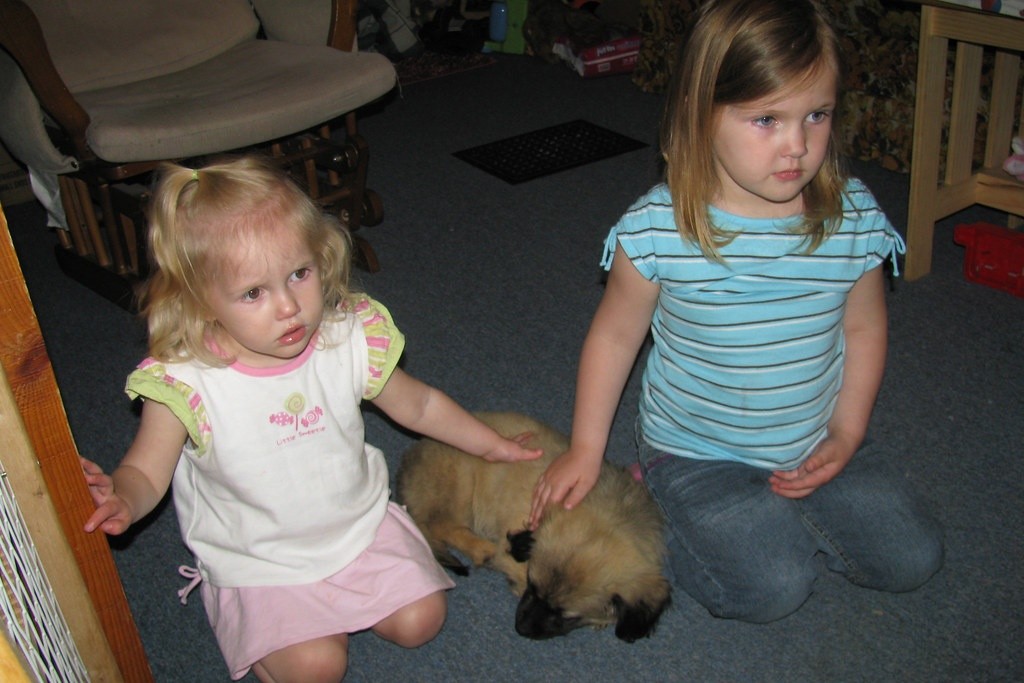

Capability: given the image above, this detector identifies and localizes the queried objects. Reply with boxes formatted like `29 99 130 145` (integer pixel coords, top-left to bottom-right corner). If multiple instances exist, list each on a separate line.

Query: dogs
390 412 674 643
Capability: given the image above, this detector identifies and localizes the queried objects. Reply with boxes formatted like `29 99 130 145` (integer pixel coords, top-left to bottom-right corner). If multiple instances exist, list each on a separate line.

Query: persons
78 158 545 683
525 0 946 624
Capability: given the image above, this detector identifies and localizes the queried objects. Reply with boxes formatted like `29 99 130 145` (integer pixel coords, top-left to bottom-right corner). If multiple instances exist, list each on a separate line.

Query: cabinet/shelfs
899 0 1024 283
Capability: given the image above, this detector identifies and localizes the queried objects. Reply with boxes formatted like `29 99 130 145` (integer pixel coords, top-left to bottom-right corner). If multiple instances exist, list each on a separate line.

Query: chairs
0 0 395 311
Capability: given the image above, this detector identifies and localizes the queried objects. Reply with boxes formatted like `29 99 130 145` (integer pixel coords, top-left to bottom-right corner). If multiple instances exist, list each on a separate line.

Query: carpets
395 51 499 86
451 117 652 188
